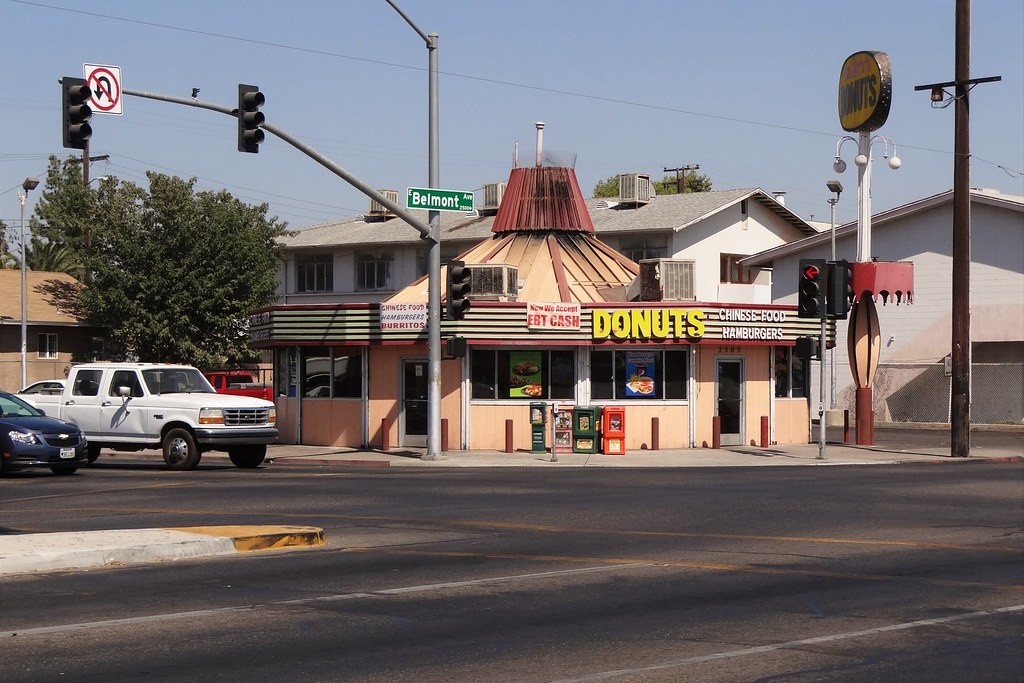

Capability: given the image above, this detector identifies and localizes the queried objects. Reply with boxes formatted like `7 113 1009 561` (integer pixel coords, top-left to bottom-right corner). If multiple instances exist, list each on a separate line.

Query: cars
0 389 89 478
174 371 196 391
12 378 100 397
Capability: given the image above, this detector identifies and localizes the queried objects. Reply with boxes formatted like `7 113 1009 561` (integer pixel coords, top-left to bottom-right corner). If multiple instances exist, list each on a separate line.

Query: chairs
111 380 135 396
151 382 164 393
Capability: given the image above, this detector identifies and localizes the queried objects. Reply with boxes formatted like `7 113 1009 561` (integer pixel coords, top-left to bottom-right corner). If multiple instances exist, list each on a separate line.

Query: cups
637 365 647 376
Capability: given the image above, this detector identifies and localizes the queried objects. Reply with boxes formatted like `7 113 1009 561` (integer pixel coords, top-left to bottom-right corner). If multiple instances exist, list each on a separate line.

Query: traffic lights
799 259 826 322
238 84 265 156
446 259 473 321
62 75 93 149
448 337 468 357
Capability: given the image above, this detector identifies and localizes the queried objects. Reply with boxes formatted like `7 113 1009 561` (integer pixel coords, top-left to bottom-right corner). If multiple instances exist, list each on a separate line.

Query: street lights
827 180 844 408
19 176 41 388
80 177 108 283
836 134 902 444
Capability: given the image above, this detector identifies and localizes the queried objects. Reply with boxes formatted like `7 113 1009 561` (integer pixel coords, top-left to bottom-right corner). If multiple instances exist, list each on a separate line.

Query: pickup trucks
0 362 280 471
200 371 274 402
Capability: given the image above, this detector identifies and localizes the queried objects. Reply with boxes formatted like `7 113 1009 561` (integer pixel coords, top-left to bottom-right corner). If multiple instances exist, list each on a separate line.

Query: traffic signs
408 186 475 212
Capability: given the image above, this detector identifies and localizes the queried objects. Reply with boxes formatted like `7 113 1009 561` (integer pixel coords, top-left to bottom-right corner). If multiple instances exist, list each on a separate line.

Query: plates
510 377 527 388
512 364 540 376
638 377 654 394
521 387 542 397
